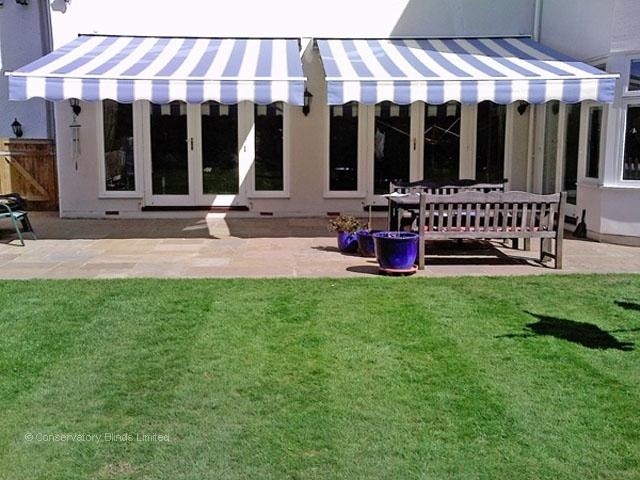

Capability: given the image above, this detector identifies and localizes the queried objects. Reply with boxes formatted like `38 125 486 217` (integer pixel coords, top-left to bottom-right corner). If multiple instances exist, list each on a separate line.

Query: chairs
0 192 38 246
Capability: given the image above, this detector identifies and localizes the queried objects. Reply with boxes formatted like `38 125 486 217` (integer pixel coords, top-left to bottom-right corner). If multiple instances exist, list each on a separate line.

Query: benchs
387 177 570 271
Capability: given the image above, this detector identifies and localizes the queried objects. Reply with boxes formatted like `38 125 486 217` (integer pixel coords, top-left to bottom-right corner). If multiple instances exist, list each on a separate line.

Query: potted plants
328 215 364 253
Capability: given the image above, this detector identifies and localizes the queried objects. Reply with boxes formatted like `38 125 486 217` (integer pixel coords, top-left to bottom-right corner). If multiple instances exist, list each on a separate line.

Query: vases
359 228 419 275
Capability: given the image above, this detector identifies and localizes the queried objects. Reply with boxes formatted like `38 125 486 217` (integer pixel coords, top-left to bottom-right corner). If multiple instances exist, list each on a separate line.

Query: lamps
11 116 24 137
516 102 530 115
301 86 313 116
69 98 84 116
